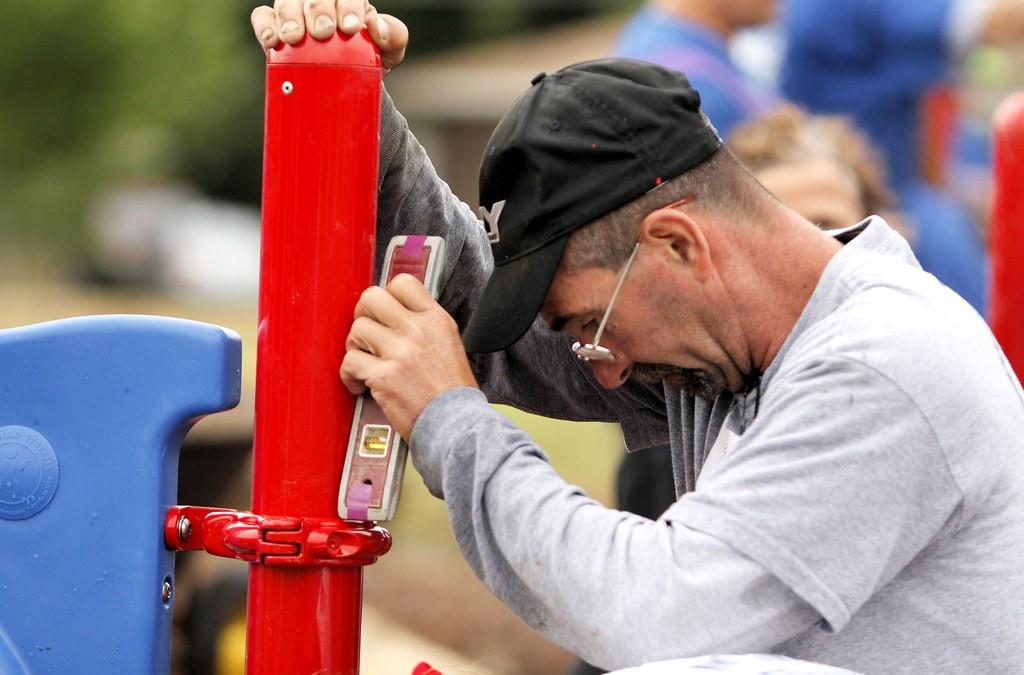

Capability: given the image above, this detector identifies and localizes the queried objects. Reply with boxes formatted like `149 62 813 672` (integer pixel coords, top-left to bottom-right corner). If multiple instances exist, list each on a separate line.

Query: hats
460 55 724 356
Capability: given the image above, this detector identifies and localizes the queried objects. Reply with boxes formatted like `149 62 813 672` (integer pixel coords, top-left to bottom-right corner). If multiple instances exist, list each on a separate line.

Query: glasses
568 190 700 363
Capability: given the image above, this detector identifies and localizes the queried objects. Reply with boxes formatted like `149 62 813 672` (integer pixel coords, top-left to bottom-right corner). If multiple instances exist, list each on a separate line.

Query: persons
252 0 1024 675
